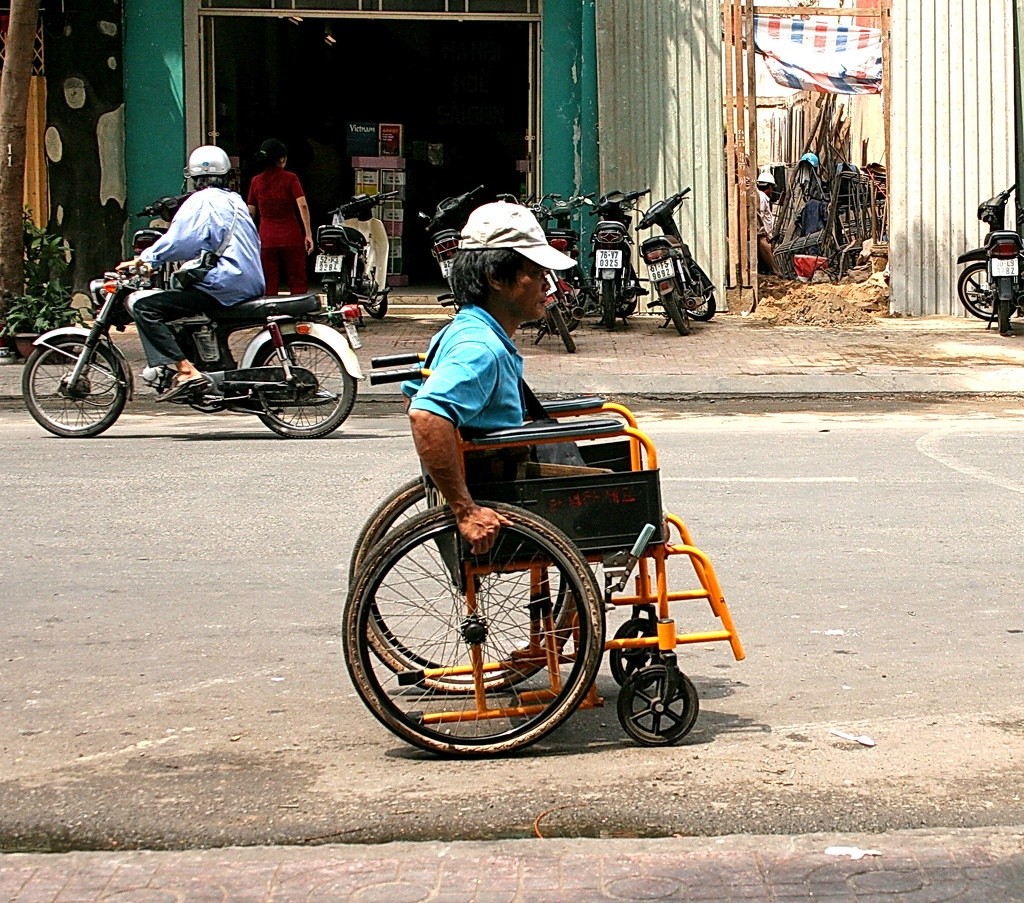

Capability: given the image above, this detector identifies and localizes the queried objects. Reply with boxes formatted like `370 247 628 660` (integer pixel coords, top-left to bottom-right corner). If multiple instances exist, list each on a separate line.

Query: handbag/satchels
332 208 345 227
521 419 587 468
170 249 218 290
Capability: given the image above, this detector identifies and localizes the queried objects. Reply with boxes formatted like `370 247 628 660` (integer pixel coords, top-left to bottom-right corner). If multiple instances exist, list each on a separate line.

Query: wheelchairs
340 356 745 762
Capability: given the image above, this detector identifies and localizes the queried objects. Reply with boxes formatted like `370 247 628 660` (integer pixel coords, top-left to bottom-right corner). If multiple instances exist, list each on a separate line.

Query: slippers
153 376 207 402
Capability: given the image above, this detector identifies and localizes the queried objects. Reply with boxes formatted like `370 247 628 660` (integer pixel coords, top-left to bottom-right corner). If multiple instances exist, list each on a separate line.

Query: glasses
517 268 549 280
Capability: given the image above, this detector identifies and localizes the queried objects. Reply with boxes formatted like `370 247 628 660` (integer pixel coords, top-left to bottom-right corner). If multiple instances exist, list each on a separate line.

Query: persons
246 139 315 295
114 145 268 403
401 199 591 557
756 172 788 276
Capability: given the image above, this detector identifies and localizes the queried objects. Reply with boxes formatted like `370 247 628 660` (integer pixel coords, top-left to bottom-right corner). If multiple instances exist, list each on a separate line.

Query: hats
458 201 578 270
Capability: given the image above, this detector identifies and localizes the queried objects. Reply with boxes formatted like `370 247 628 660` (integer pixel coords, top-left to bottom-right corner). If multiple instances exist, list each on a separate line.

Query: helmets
757 173 775 186
189 145 231 177
799 152 818 168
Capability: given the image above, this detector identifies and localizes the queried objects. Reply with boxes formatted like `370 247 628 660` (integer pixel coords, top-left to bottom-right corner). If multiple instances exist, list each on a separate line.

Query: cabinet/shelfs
351 156 409 286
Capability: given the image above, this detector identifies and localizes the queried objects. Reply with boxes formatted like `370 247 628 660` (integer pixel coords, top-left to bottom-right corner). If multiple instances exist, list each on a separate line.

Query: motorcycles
20 259 366 441
418 184 484 316
132 165 192 294
954 183 1024 334
576 188 653 331
496 190 595 355
306 190 399 333
618 187 718 337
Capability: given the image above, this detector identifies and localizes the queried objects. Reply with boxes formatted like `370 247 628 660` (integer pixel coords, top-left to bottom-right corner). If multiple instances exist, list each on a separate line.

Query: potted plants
0 202 100 365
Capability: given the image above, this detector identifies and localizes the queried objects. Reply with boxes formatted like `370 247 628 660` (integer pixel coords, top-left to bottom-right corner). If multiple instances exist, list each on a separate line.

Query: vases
0 324 17 365
